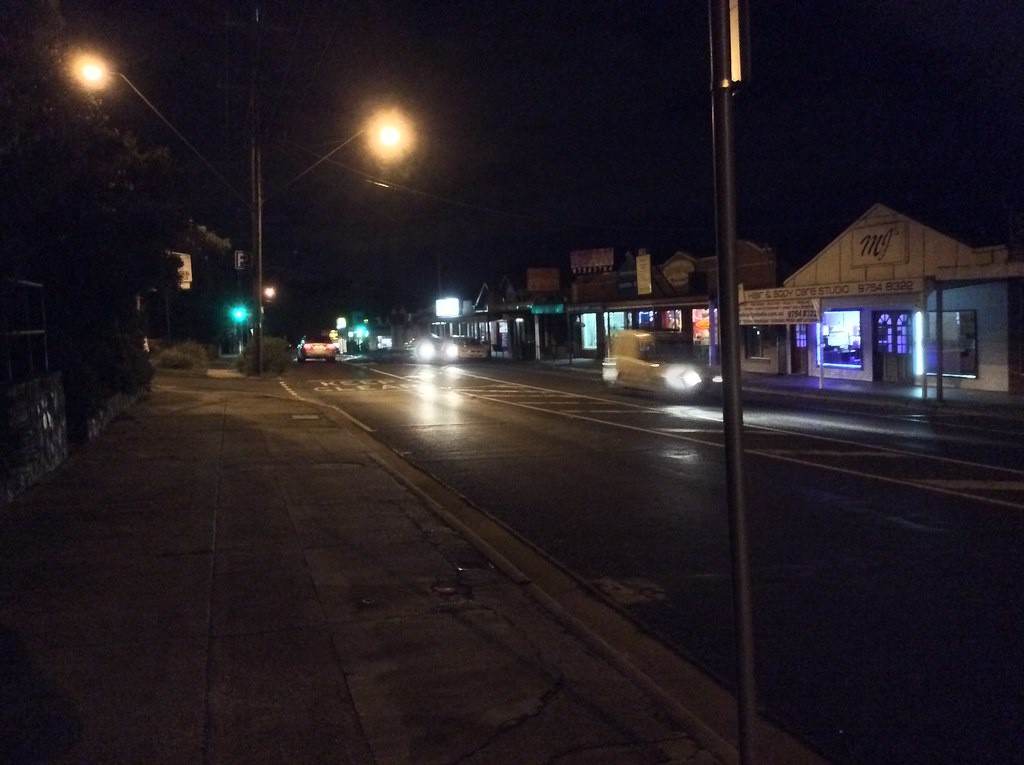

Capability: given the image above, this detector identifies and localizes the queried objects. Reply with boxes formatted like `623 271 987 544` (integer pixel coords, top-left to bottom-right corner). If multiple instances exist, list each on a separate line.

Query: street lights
77 54 413 378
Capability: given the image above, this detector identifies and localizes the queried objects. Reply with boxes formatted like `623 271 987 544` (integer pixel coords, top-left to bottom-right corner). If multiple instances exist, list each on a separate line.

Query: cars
297 334 336 362
601 329 724 400
405 334 459 366
442 336 488 364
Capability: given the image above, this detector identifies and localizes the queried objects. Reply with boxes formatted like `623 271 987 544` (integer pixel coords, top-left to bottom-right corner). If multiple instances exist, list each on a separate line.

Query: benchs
492 344 507 360
